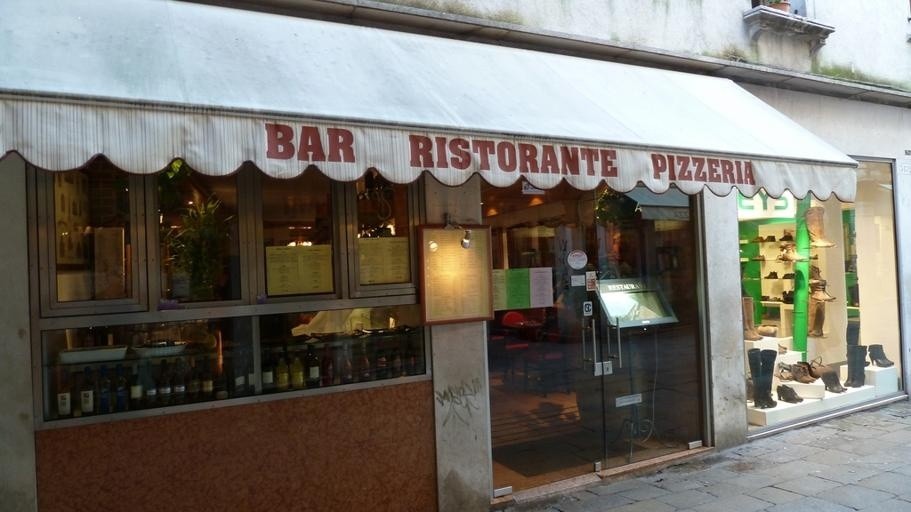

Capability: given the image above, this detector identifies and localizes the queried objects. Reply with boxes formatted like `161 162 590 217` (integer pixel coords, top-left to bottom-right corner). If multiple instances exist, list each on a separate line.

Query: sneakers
751 235 793 241
764 271 778 279
761 290 794 304
752 255 764 261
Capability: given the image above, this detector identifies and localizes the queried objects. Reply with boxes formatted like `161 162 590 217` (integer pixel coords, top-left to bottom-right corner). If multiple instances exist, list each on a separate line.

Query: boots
743 296 763 341
806 206 836 337
844 255 869 388
749 348 777 408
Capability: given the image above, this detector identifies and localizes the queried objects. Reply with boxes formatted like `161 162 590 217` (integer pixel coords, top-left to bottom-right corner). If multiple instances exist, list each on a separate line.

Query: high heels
776 362 819 383
778 384 803 403
869 344 894 366
822 370 847 393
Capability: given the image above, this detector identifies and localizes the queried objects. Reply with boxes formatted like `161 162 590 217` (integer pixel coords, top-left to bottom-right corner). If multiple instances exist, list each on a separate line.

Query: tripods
611 330 662 463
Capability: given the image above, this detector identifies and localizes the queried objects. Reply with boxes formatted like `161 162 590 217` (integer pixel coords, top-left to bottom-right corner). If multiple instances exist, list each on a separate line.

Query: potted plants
767 0 792 14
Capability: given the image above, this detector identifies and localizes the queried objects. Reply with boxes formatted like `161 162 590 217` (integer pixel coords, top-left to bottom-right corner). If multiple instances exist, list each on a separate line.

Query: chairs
484 311 573 399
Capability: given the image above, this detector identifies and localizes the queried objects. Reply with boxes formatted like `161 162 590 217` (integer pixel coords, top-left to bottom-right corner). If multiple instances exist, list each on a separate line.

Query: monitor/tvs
594 277 679 330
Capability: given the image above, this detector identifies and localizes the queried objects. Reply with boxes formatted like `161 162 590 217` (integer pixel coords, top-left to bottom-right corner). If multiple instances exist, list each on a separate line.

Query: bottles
53 323 426 421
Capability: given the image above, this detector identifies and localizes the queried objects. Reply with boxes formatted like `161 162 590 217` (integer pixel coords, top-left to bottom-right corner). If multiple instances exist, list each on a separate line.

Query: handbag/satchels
810 358 832 376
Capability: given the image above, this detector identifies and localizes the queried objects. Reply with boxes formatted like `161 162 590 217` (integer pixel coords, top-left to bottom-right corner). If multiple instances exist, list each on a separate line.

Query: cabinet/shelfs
735 233 820 342
